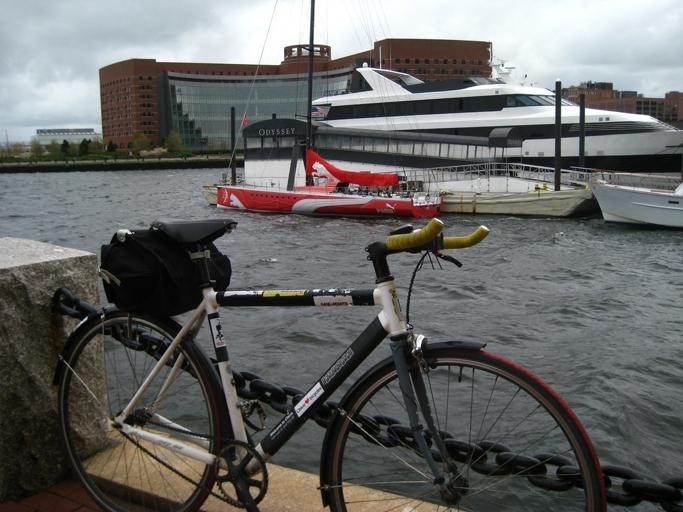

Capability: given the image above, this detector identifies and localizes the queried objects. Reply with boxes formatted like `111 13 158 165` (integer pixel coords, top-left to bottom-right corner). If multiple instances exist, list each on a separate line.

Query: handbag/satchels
97 228 232 318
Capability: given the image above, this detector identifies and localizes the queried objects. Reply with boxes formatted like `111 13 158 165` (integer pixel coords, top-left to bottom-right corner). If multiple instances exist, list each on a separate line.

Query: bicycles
50 210 610 511
